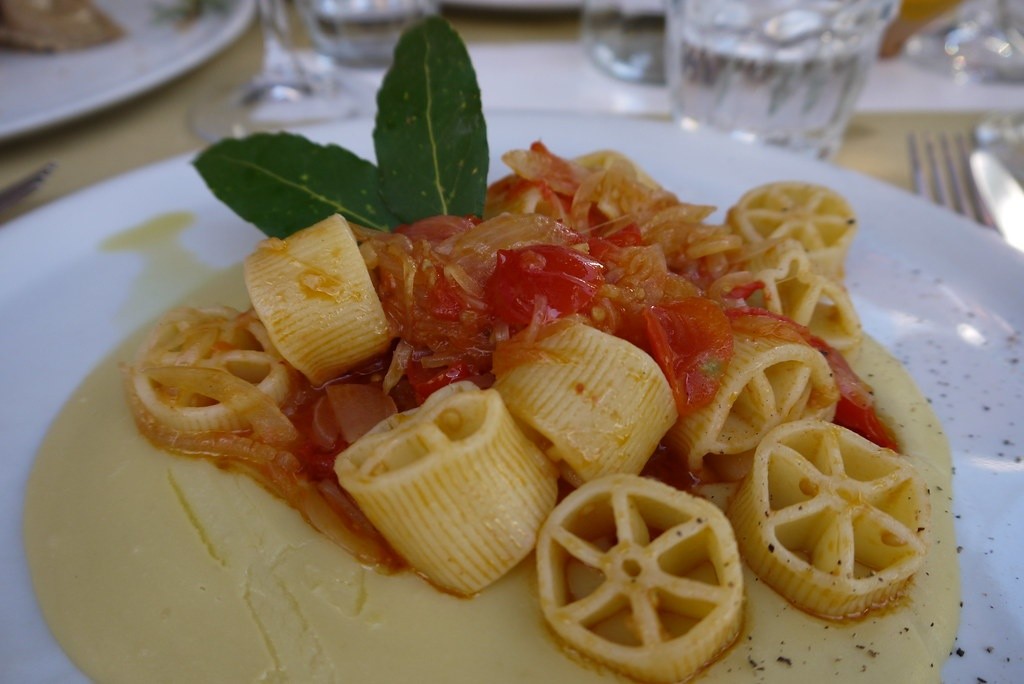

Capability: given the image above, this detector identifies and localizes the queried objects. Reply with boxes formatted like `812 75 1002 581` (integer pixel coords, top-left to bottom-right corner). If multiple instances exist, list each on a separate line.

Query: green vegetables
371 15 489 226
190 132 407 239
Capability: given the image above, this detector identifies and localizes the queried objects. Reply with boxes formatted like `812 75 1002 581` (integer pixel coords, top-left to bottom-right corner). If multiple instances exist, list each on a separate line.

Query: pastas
129 181 932 684
